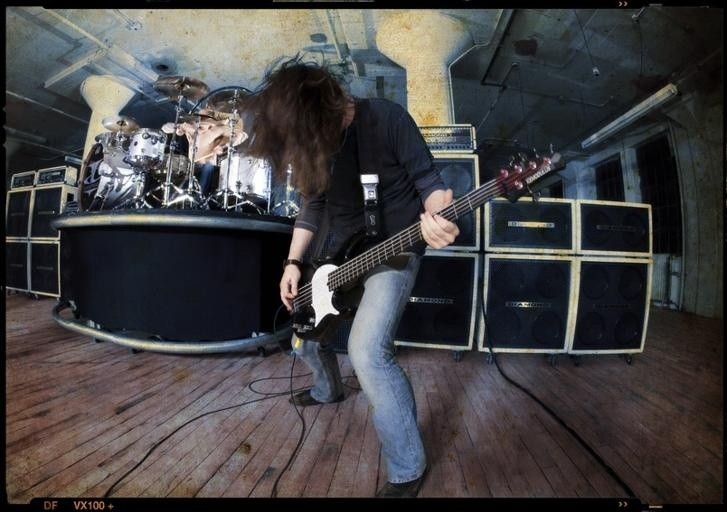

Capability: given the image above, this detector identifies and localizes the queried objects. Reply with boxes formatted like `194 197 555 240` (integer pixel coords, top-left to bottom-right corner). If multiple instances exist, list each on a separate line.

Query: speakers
29 240 61 298
29 185 79 240
5 188 33 240
6 239 29 292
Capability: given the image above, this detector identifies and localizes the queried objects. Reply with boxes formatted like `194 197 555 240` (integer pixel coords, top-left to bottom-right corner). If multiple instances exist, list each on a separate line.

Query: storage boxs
3 182 80 301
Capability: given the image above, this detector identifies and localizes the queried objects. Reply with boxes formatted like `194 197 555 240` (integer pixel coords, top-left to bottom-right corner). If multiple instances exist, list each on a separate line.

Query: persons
180 116 231 201
242 50 461 497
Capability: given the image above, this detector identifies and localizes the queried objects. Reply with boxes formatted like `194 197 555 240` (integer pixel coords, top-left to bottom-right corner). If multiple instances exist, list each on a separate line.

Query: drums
80 143 145 212
214 152 272 204
126 128 167 170
150 153 190 184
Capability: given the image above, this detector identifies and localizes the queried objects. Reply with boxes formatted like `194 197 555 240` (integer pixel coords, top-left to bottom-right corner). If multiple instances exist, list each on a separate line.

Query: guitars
287 144 565 339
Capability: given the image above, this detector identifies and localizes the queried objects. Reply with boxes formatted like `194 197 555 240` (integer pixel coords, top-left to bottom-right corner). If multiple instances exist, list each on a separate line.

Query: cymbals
102 115 137 133
153 76 210 101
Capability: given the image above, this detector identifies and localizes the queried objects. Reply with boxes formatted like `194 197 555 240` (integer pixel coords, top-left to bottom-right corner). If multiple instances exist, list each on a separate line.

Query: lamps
62 154 84 166
4 124 48 149
41 40 111 92
575 11 679 157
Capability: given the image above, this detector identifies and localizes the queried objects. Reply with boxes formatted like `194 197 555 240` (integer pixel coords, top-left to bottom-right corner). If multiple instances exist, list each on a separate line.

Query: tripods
93 94 301 220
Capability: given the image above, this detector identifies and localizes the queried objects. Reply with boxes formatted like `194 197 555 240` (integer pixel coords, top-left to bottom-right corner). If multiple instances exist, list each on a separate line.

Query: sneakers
374 471 427 497
289 389 346 405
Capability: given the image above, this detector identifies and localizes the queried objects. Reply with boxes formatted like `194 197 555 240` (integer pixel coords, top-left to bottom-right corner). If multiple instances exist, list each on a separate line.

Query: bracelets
281 258 302 269
